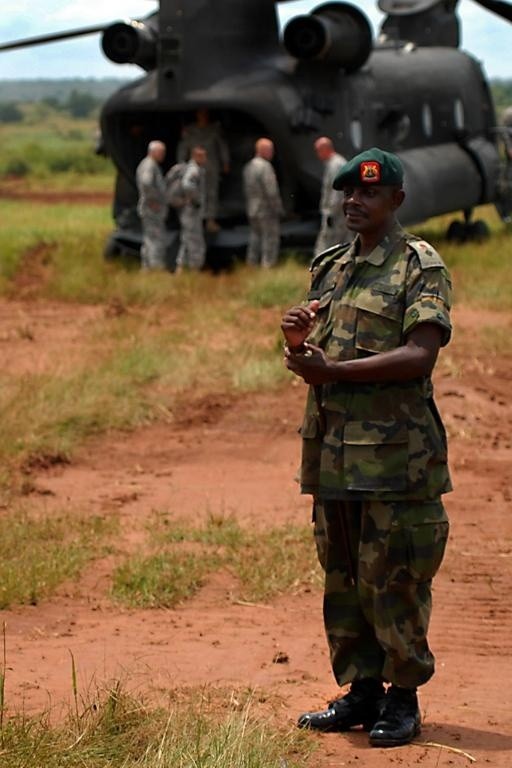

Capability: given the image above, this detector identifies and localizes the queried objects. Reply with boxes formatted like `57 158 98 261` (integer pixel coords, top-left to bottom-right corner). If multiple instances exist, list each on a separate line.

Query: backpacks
163 164 202 208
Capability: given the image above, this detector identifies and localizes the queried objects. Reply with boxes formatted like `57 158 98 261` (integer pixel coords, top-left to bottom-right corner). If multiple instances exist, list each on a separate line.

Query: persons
173 145 210 274
134 139 172 274
281 146 457 748
241 137 303 269
176 108 233 233
313 135 356 259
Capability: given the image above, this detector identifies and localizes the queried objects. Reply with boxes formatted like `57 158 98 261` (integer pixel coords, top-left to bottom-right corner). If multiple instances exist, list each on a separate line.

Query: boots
299 678 385 733
370 687 421 746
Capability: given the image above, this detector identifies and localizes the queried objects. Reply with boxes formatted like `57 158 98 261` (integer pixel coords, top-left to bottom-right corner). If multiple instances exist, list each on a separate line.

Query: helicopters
0 1 512 275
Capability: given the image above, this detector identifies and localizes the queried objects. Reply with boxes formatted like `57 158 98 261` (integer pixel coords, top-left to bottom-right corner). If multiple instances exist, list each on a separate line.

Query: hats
331 147 404 190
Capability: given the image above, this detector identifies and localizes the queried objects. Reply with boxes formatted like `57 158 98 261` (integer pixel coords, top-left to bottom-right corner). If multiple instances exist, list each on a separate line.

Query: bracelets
286 340 307 354
